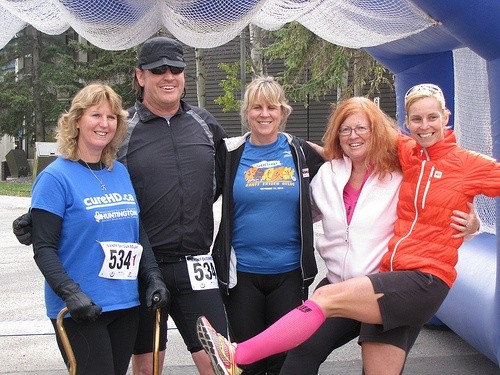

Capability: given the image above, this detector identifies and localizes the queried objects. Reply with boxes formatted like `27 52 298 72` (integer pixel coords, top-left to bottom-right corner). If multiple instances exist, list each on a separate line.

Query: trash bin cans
5 149 30 178
33 141 58 185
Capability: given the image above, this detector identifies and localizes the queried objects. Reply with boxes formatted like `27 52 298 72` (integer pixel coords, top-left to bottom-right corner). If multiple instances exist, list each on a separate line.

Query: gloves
144 270 172 320
57 283 102 325
12 213 33 246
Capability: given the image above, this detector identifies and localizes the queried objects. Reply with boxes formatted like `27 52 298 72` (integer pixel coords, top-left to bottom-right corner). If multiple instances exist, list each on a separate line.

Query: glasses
148 64 185 75
404 84 444 116
337 125 370 136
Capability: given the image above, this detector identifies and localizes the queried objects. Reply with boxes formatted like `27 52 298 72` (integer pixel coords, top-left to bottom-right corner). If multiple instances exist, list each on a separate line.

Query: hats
137 36 187 69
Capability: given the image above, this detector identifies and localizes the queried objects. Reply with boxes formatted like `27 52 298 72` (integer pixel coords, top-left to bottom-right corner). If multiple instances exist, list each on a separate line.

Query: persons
276 96 484 375
197 84 500 375
13 36 235 375
29 82 171 375
211 73 327 375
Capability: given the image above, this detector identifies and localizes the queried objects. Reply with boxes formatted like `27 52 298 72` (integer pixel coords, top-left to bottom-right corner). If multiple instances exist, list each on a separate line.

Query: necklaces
77 146 107 191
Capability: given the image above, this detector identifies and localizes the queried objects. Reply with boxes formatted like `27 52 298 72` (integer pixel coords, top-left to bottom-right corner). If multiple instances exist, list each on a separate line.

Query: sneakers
196 315 243 375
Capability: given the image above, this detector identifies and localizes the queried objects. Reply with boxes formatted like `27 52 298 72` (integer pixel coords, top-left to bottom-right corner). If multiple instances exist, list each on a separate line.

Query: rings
465 222 470 228
